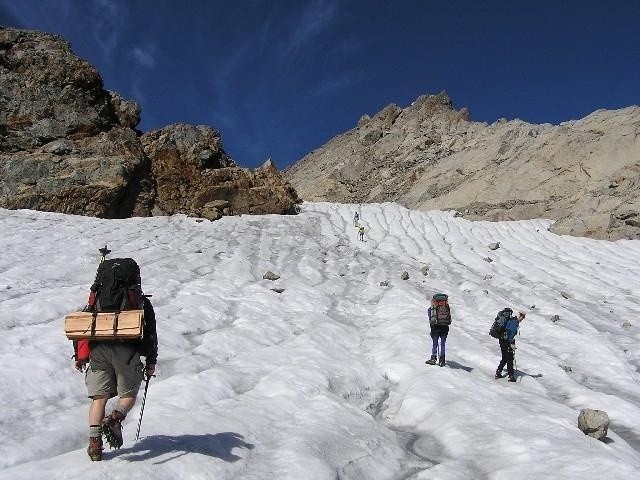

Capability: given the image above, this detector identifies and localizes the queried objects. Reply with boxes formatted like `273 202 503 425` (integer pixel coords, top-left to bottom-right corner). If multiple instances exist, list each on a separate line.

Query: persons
71 289 158 461
493 312 526 382
358 225 365 241
354 212 360 227
426 305 453 366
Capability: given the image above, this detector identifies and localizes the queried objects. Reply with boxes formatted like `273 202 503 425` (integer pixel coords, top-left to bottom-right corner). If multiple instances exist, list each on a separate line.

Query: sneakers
426 357 436 365
439 361 445 367
87 443 102 461
102 415 123 447
496 373 503 378
509 374 516 382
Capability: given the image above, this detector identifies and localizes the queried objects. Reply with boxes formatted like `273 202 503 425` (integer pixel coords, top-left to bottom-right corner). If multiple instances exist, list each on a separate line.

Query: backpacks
430 293 452 327
489 308 513 338
89 257 143 314
